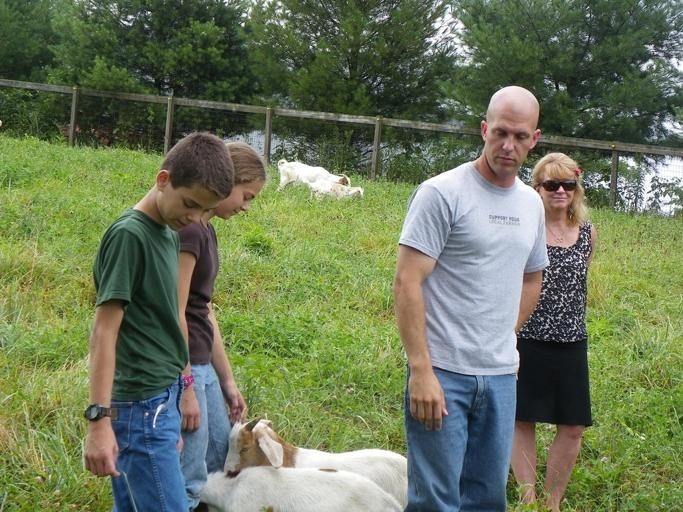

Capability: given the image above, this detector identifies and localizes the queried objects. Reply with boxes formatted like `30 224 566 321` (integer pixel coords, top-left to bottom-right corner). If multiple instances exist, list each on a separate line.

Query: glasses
535 178 577 192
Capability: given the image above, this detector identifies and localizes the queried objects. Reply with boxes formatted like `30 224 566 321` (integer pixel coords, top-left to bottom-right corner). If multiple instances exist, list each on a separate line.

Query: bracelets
178 374 193 390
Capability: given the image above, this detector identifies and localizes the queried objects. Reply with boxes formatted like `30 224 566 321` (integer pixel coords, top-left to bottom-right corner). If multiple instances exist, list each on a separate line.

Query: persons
390 83 552 512
79 131 238 511
509 151 598 512
171 139 268 511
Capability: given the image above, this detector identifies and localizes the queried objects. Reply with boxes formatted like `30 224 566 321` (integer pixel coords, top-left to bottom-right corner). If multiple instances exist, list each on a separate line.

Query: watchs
79 404 112 421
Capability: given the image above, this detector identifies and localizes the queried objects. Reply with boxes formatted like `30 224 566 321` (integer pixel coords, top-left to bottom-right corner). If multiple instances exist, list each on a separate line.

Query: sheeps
309 180 363 203
273 159 350 205
223 407 408 512
184 468 405 512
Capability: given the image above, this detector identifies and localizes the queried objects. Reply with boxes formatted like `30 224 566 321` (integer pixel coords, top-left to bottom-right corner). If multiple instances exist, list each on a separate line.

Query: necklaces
545 215 571 244
